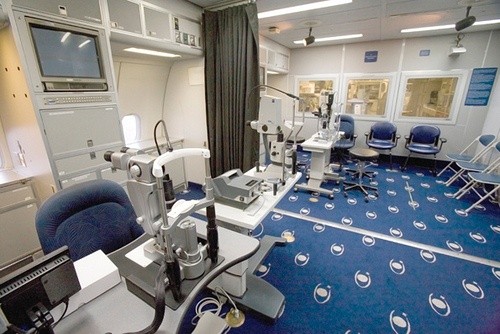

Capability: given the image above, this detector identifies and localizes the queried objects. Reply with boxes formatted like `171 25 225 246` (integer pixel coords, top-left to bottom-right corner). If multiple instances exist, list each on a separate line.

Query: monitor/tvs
0 244 81 331
23 15 108 92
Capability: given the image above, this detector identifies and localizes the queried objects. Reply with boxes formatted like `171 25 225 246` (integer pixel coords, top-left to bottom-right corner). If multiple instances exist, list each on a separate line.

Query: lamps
302 27 315 47
448 33 466 55
268 27 280 35
455 5 476 31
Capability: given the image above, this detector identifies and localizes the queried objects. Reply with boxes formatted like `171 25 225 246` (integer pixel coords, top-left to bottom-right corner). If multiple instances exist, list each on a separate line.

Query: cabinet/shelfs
0 168 43 281
125 136 190 194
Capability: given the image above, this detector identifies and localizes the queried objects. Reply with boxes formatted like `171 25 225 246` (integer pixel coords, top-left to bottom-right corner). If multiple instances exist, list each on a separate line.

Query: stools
343 146 380 204
285 135 308 171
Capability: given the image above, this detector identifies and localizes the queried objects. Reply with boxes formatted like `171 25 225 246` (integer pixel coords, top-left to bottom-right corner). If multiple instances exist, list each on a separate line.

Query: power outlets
19 154 26 168
49 184 56 195
14 151 22 168
225 309 246 328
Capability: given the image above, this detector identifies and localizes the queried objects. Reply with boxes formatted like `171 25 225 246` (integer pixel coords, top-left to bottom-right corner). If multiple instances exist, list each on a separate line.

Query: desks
0 128 346 334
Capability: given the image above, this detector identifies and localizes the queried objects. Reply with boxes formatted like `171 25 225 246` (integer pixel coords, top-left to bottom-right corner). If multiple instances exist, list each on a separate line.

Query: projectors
448 47 468 55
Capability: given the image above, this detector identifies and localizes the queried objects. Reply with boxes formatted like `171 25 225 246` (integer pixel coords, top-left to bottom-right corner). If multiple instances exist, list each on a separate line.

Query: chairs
34 179 145 263
321 115 358 166
400 124 447 176
437 132 500 213
364 120 402 171
